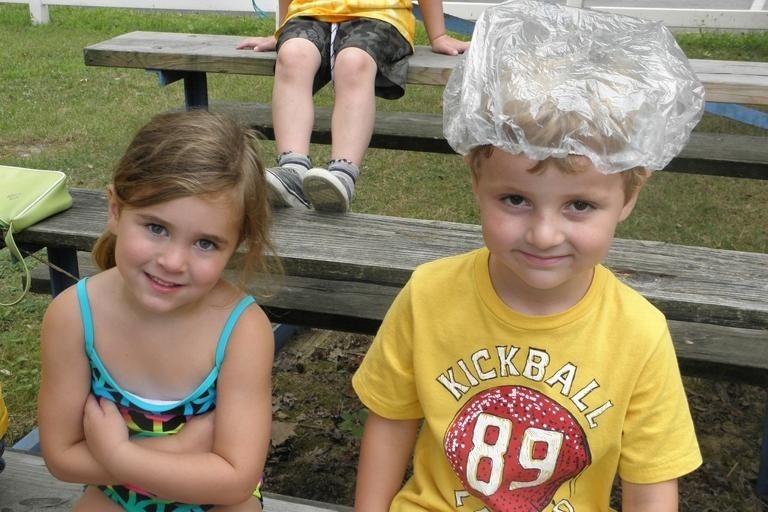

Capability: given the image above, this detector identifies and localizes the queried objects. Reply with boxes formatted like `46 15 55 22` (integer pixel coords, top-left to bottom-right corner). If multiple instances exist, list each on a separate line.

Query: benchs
0 447 348 509
16 185 766 403
83 29 768 182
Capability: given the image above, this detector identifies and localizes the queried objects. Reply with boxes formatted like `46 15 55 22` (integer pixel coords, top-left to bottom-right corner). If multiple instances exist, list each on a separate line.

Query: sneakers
264 161 309 210
302 169 350 212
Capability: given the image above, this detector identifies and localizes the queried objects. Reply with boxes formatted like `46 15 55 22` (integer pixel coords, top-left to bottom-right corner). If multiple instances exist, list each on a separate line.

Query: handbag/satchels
0 165 74 306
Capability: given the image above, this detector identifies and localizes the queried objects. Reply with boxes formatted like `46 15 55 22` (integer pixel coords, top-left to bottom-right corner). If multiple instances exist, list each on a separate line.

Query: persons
236 0 470 213
36 110 285 512
353 60 703 512
